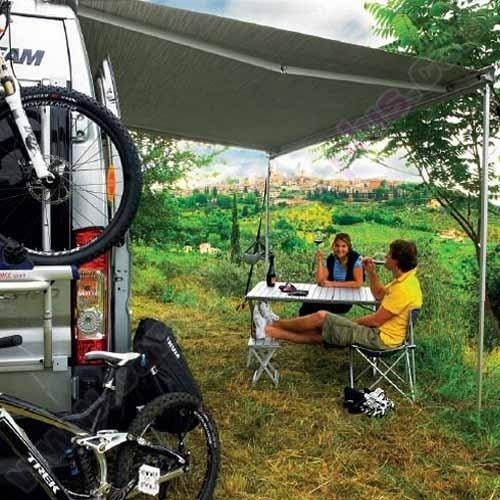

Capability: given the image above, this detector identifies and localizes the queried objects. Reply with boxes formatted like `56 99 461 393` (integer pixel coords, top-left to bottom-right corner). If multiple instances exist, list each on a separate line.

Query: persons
251 240 424 352
299 232 365 319
198 239 211 255
182 241 194 254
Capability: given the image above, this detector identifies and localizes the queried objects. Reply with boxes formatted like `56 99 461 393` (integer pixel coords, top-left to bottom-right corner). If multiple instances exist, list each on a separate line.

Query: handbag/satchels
133 318 203 434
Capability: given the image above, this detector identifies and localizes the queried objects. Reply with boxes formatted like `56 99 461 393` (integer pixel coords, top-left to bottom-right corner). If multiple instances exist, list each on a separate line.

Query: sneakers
252 300 279 340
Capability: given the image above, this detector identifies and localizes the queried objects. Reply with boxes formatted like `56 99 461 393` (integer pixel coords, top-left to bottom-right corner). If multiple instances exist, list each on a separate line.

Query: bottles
266 255 276 286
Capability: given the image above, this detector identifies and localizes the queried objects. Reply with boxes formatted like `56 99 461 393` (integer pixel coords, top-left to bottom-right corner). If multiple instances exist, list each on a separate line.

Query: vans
0 0 132 421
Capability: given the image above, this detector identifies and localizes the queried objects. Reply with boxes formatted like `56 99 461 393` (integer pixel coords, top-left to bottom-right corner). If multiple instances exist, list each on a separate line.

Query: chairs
349 307 421 407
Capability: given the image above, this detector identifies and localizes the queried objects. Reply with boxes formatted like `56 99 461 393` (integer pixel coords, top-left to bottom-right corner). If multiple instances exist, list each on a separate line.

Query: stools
248 336 281 390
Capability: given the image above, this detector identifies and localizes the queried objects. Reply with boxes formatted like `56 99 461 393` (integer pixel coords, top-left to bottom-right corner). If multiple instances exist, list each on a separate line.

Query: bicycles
0 350 221 500
0 0 145 270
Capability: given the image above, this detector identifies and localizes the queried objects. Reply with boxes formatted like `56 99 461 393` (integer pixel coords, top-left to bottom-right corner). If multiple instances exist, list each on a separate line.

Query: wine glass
358 251 387 264
314 232 324 256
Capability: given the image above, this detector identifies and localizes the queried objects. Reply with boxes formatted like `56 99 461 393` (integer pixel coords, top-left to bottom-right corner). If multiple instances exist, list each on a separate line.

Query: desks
245 280 380 381
